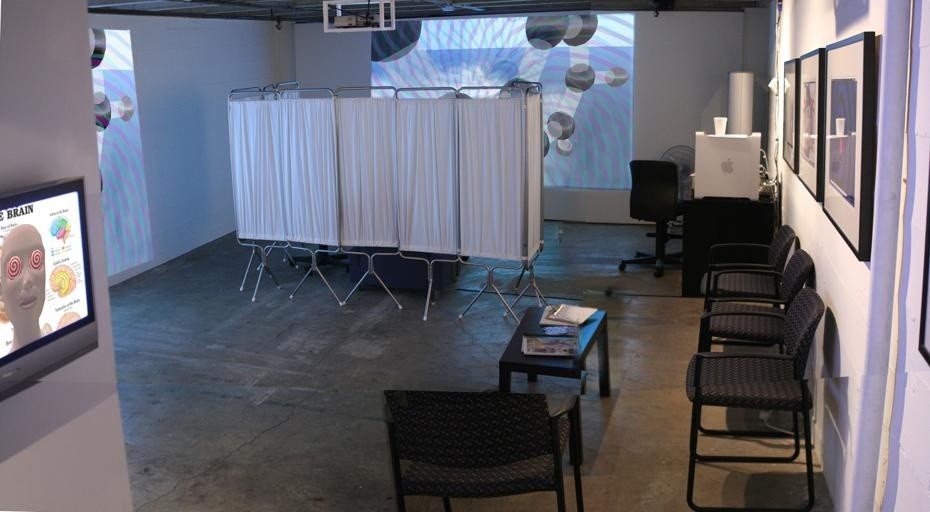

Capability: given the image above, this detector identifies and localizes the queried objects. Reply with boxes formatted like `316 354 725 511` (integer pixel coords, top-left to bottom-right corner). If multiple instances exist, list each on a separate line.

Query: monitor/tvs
0 174 99 402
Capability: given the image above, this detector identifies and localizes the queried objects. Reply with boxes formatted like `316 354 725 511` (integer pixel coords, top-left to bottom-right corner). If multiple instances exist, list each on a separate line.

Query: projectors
333 13 380 29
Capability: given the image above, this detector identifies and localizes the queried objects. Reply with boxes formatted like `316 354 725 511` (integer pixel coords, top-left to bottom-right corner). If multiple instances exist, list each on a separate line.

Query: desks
681 193 778 299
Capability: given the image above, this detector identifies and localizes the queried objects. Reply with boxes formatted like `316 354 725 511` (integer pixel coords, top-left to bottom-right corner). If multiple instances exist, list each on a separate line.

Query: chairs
619 159 685 278
685 288 826 511
694 248 815 437
703 225 795 347
384 390 584 512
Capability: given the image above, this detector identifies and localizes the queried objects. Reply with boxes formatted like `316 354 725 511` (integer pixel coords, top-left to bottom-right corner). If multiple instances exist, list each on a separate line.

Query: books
519 333 580 358
538 303 599 328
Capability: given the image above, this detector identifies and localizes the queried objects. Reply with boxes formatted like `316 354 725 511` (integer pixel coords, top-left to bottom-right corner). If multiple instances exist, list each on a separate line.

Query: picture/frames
823 31 877 263
797 47 826 203
783 57 800 174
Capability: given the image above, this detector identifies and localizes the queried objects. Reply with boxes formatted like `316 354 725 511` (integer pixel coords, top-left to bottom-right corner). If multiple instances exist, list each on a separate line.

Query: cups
713 117 727 136
835 118 845 135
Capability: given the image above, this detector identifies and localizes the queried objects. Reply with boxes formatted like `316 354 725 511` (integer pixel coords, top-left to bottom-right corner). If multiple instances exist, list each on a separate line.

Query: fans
658 144 695 202
